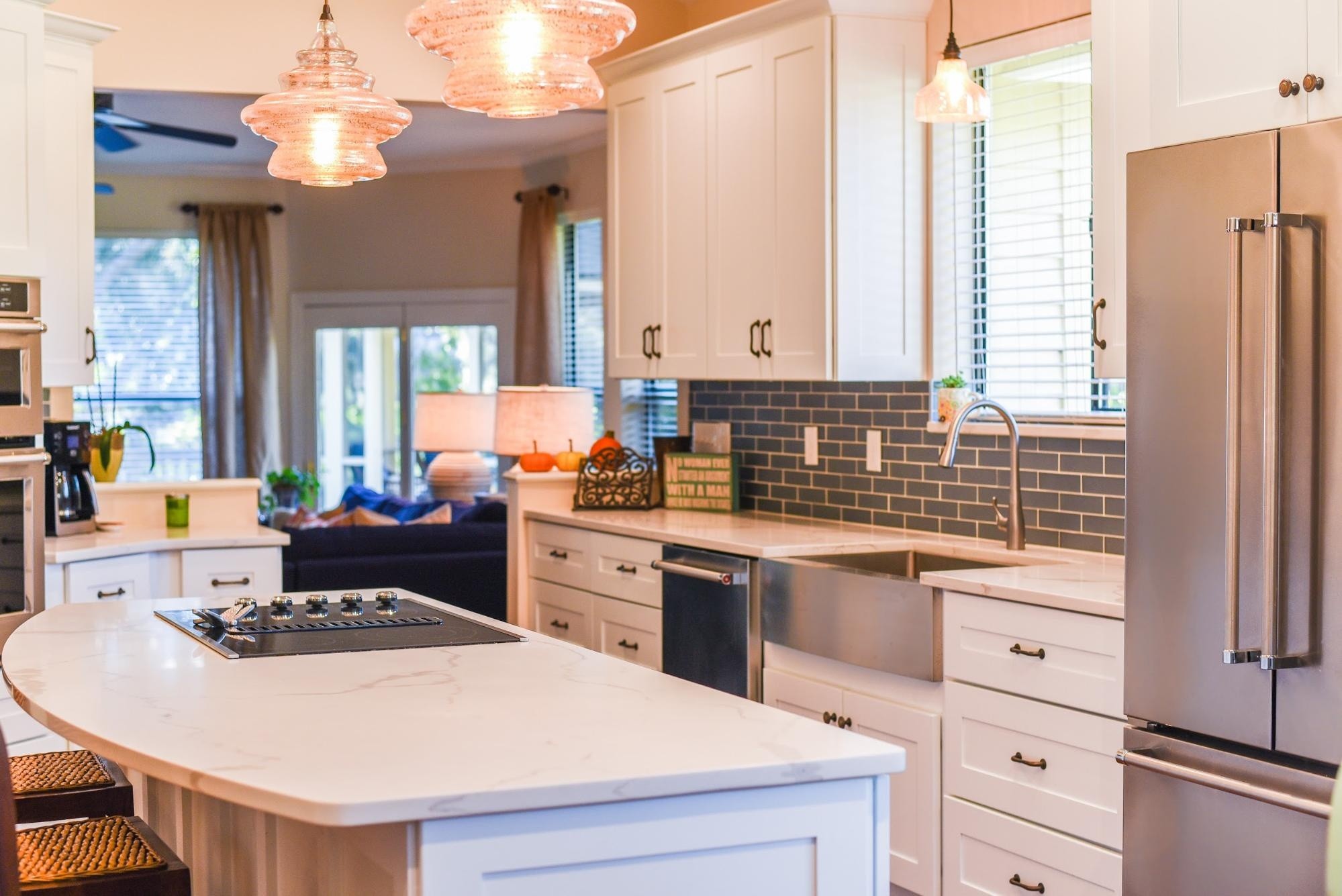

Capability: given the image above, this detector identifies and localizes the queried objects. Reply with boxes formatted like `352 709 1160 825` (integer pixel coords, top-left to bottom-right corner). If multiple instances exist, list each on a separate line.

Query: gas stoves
153 591 529 660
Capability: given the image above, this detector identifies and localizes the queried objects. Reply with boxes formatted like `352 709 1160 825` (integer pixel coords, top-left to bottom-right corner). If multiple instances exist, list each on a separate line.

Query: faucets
936 400 1029 552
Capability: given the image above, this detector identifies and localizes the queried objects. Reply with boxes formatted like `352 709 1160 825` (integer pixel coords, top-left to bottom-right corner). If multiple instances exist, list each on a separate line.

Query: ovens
0 274 53 661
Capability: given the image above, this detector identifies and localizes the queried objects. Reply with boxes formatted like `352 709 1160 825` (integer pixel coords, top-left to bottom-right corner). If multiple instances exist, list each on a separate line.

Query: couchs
271 485 508 622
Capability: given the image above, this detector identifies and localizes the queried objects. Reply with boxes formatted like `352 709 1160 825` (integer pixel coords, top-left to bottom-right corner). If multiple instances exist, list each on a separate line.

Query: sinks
760 548 1071 684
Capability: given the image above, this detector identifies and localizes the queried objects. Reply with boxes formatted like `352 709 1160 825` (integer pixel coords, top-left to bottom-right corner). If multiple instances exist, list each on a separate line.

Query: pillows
287 486 482 530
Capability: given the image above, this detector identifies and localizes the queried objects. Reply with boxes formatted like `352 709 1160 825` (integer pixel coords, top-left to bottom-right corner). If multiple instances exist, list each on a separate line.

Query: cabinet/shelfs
1089 0 1342 378
0 0 117 385
527 520 662 668
590 0 926 383
64 546 277 603
762 591 1124 896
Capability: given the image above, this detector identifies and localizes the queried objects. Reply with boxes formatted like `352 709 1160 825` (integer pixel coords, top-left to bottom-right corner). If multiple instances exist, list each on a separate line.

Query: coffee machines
42 418 100 538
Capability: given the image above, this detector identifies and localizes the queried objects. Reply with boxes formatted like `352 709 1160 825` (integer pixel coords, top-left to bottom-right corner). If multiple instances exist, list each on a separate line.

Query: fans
94 93 237 154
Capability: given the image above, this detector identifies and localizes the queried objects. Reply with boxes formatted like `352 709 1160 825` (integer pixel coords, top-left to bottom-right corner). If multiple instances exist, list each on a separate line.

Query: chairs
0 724 192 896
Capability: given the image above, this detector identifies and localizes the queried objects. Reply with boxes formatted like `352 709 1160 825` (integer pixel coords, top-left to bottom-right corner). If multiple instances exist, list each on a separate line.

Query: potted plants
939 375 984 423
85 356 156 483
267 463 320 509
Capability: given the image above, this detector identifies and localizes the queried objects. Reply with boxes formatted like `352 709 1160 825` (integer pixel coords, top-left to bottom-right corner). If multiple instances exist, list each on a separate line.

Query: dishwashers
650 541 762 705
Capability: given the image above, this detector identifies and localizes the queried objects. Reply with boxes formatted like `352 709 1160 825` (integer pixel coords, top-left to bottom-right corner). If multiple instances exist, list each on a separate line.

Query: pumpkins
519 441 555 472
555 439 587 471
589 430 625 470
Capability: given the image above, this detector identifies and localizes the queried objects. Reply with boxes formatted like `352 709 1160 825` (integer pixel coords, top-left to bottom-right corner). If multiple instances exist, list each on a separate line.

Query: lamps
403 0 637 120
412 391 494 452
493 383 595 456
916 0 989 122
241 0 412 187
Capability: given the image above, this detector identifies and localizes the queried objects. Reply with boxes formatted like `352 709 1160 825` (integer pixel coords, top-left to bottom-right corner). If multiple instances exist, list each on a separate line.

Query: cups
165 493 190 527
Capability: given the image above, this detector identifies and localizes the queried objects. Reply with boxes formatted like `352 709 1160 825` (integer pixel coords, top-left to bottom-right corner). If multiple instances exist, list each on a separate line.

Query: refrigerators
1115 117 1342 896
